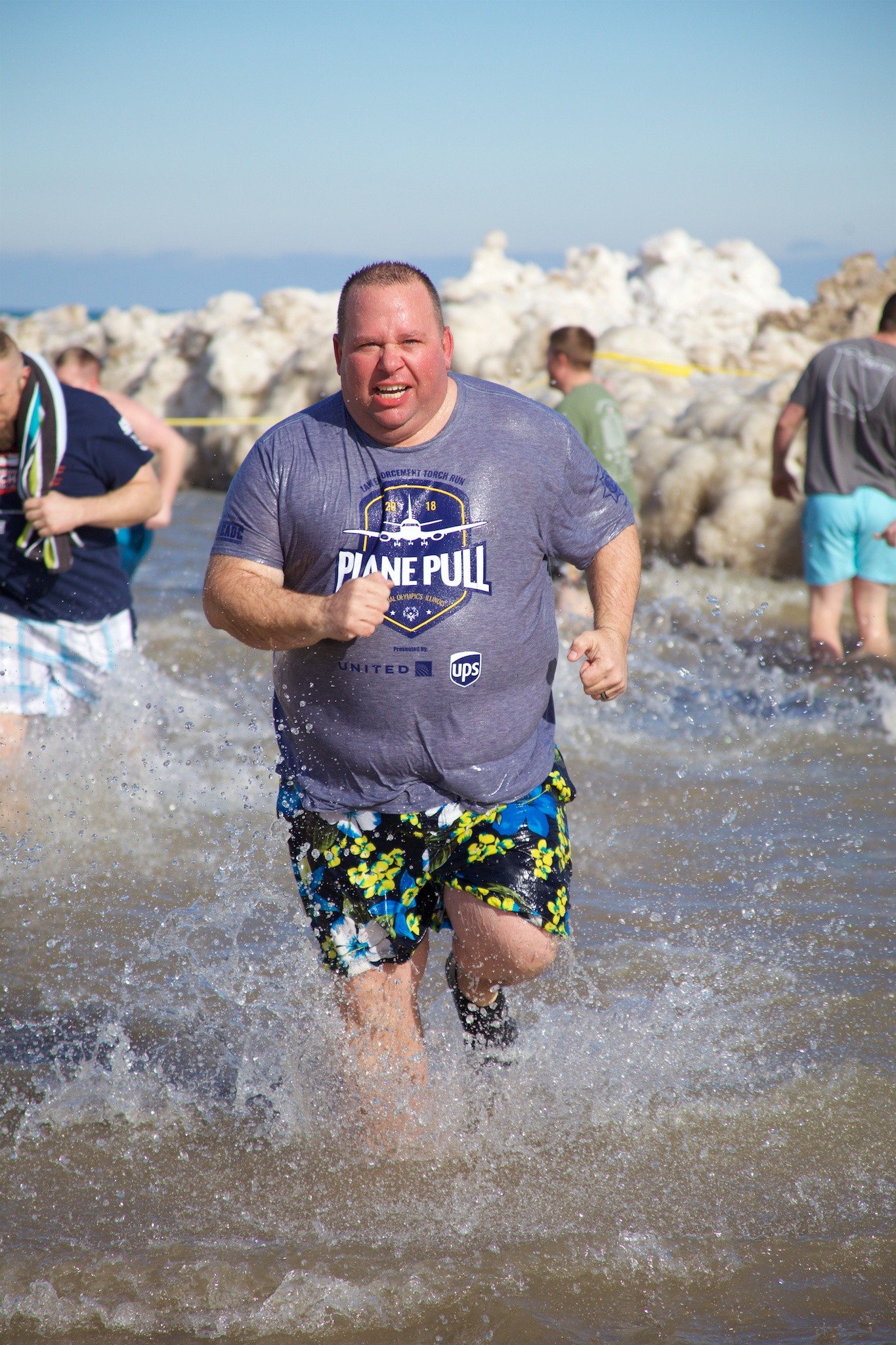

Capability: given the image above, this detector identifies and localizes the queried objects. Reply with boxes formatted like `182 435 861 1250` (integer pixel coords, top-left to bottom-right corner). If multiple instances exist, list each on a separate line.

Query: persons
0 332 162 748
546 326 637 521
200 260 644 1075
770 289 896 658
54 348 194 635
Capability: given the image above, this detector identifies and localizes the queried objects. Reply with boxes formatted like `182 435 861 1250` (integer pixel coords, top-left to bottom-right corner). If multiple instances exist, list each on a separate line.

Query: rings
601 691 609 699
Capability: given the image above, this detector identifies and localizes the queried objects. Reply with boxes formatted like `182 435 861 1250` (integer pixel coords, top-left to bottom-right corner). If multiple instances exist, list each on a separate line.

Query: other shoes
445 950 520 1070
